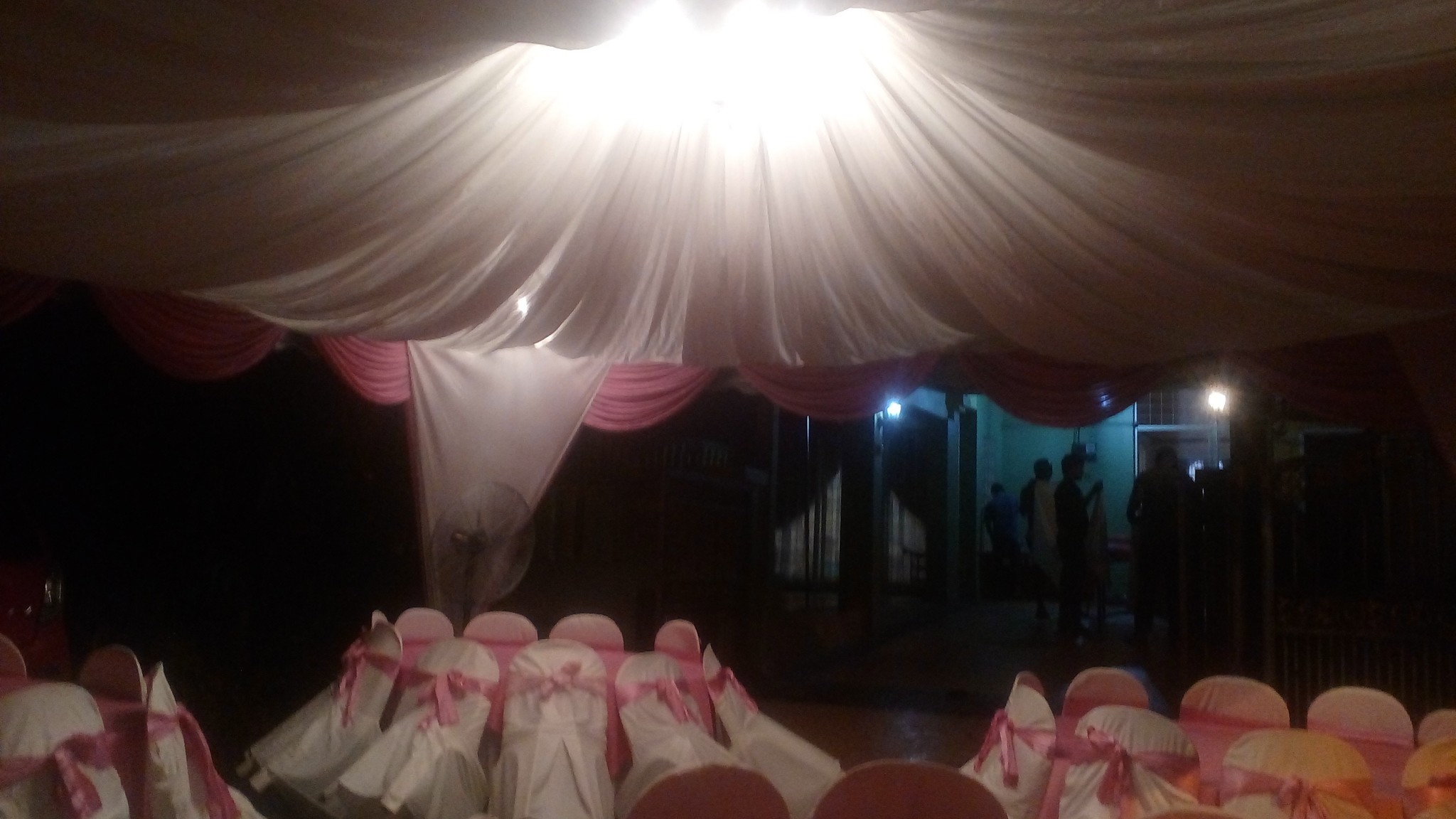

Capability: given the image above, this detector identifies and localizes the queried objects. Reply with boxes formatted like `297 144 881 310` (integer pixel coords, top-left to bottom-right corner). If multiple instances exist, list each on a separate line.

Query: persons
979 442 1215 630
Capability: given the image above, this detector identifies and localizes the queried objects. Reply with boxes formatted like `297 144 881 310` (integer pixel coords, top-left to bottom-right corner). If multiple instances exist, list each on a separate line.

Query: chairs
0 595 1456 819
